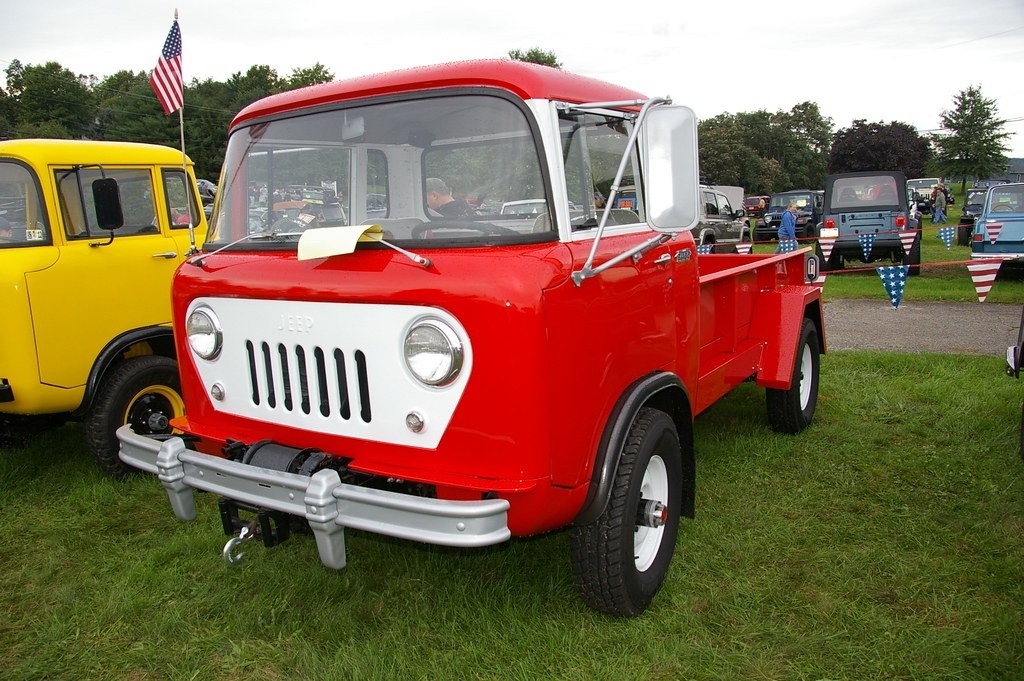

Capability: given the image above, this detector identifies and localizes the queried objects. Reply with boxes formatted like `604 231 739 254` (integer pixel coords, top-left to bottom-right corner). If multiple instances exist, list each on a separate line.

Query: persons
426 177 464 218
176 204 190 224
0 217 13 243
774 202 799 254
930 182 948 224
250 184 301 207
758 199 767 218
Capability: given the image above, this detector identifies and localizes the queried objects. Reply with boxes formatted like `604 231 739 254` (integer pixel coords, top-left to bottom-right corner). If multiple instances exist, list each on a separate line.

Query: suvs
907 178 941 215
498 199 594 213
611 183 825 260
249 186 387 232
958 180 1024 280
817 172 921 275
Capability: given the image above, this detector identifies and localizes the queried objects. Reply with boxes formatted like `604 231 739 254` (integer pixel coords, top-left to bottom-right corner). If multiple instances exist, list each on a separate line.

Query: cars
1 138 216 484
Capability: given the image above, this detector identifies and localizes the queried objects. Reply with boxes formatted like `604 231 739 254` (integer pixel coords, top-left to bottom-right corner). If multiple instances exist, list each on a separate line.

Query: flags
149 20 184 115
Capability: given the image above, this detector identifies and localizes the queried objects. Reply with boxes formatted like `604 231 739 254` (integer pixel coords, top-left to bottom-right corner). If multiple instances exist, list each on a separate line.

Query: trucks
116 59 827 619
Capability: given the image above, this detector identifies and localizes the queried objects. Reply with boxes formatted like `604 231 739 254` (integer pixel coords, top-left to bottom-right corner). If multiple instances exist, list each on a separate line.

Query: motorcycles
945 186 955 204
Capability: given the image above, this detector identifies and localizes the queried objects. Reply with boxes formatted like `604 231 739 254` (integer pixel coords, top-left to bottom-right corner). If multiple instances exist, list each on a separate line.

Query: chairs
878 185 895 199
363 219 425 241
839 188 858 203
533 210 639 232
783 198 791 205
